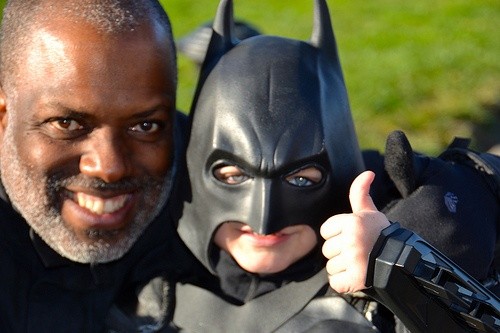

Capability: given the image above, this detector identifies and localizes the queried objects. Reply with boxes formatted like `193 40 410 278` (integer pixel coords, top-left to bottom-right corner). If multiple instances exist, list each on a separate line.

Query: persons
115 0 500 333
0 0 500 333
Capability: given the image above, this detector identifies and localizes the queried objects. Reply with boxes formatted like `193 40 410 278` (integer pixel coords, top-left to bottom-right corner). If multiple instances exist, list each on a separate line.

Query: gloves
383 131 500 281
